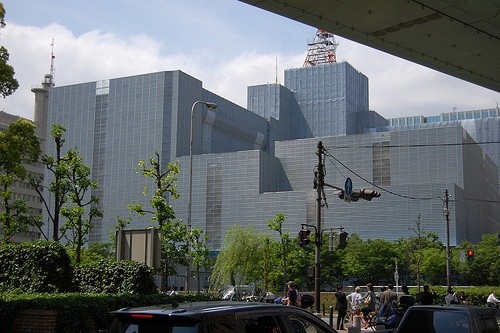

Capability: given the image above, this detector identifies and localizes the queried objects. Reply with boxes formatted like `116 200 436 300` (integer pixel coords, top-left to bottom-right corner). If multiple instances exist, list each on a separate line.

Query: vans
221 284 259 301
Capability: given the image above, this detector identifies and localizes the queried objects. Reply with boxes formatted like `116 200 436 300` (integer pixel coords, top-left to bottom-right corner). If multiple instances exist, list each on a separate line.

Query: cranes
301 27 339 68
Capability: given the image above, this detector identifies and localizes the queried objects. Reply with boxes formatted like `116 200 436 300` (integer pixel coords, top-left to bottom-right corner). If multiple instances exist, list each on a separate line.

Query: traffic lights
360 188 382 201
337 232 349 250
299 229 311 247
467 250 474 262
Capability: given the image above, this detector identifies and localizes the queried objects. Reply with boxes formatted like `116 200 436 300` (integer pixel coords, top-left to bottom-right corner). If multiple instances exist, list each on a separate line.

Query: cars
98 300 338 333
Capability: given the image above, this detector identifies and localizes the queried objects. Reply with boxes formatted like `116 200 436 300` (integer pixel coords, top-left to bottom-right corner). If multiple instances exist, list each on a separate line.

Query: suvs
393 304 500 333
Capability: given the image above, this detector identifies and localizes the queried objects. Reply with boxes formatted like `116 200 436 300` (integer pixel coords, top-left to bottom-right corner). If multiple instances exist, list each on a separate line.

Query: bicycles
342 300 378 332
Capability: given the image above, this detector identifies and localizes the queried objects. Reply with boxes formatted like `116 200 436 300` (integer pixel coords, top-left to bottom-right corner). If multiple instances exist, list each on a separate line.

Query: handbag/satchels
451 295 460 304
363 291 371 305
344 312 350 323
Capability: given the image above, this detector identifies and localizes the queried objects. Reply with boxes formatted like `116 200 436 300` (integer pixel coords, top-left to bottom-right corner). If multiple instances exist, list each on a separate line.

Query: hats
401 286 408 290
286 281 295 285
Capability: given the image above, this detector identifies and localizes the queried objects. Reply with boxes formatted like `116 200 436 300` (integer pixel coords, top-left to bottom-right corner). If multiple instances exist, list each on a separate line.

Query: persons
377 282 433 317
486 291 500 309
345 286 365 324
356 283 376 329
334 286 347 330
445 288 467 305
292 284 302 307
286 281 297 305
252 287 262 302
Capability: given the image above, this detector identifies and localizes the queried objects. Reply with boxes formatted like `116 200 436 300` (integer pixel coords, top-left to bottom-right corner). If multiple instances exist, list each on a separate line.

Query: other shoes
336 329 339 330
364 324 370 328
340 329 346 330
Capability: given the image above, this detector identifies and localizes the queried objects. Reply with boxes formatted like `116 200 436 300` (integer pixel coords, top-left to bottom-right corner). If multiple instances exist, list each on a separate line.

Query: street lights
185 100 218 291
